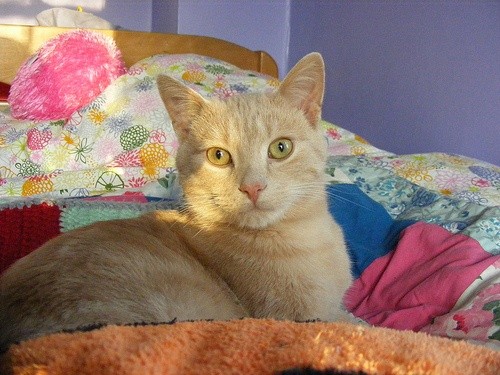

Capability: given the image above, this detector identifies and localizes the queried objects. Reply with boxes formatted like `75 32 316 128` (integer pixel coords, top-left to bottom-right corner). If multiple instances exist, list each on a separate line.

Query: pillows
7 32 123 121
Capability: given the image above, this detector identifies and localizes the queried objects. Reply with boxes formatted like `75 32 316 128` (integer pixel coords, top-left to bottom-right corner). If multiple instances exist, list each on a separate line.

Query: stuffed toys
8 29 119 121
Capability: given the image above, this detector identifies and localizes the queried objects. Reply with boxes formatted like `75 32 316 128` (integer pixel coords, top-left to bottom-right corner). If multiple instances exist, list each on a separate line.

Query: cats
0 51 355 354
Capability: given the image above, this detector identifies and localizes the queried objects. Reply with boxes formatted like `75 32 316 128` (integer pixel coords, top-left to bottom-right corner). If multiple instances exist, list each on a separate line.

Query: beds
0 25 500 351
